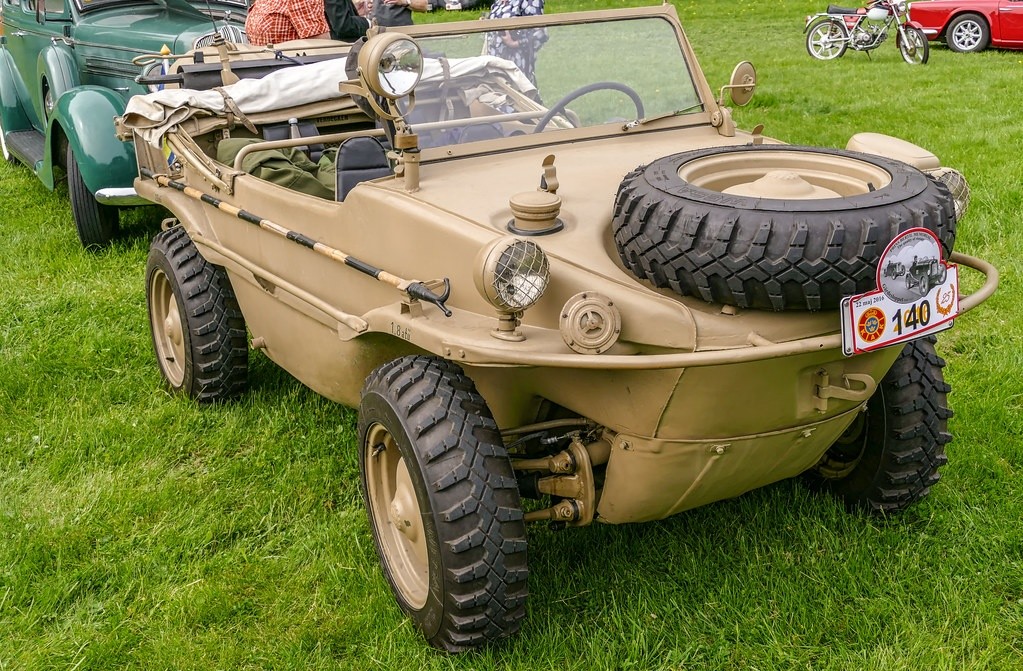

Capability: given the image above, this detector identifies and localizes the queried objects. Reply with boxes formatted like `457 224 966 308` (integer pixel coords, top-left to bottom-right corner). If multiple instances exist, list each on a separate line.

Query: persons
245 0 429 48
477 0 549 107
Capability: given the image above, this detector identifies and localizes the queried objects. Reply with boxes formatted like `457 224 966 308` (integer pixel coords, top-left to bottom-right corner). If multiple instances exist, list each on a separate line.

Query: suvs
93 3 1003 657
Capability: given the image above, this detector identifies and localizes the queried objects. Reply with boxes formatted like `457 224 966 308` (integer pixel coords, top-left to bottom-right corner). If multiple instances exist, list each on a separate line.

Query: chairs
334 135 395 202
264 121 326 165
459 122 505 143
374 87 442 148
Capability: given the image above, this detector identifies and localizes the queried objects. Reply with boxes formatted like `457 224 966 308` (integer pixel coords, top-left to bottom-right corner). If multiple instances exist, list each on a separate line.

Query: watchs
403 0 411 7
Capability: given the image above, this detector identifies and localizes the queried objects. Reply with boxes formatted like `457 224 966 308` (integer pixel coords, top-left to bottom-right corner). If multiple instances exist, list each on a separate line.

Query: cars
0 0 257 249
907 0 1023 55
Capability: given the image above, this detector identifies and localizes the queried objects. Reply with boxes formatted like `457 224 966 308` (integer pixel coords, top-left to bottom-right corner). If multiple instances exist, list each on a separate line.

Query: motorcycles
802 1 930 67
